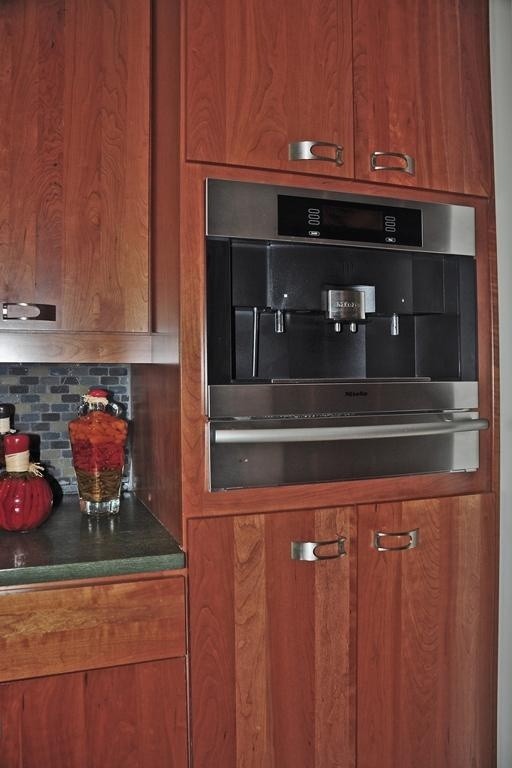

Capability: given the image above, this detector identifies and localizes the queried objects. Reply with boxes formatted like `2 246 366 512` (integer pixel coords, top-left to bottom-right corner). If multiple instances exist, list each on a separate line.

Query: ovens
203 178 491 495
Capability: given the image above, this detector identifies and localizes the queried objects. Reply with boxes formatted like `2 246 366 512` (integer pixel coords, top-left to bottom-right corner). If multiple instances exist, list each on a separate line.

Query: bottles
77 388 125 414
0 433 58 531
1 403 17 439
2 529 45 568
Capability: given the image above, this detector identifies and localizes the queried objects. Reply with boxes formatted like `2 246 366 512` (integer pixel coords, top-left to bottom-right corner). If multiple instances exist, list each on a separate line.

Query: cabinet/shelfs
184 487 499 768
0 563 187 766
3 0 158 340
182 1 499 204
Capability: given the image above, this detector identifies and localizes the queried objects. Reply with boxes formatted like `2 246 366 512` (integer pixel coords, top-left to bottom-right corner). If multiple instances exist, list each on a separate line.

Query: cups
69 421 129 515
79 512 122 550
